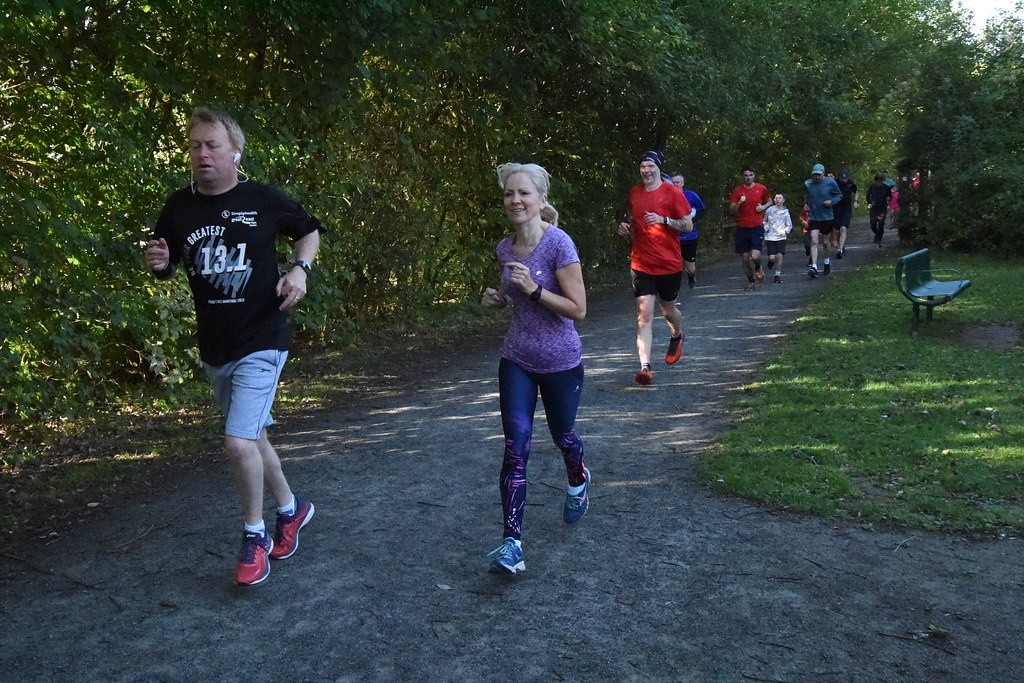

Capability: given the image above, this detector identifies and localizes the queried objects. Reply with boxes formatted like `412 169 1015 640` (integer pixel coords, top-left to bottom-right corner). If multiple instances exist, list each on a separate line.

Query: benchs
895 248 972 336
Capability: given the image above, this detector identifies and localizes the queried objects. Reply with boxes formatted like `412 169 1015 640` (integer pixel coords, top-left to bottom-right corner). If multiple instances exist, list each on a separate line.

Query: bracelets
501 294 507 308
854 200 858 202
664 216 670 225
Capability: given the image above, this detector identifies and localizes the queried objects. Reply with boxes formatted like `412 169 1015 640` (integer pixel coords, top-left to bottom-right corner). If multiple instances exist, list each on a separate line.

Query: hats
839 171 848 181
639 149 664 170
811 163 825 175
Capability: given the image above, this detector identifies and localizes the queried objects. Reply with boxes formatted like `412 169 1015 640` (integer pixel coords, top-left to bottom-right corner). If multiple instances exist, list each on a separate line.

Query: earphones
233 153 241 162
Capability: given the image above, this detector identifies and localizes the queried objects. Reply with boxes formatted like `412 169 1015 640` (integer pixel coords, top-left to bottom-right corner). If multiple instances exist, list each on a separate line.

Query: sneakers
665 331 684 365
486 537 526 574
634 368 653 386
563 463 591 524
687 275 696 289
271 495 315 560
234 527 274 586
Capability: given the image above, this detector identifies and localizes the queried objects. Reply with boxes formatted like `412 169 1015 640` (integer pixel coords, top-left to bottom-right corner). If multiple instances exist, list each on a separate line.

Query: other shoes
808 267 818 278
746 282 755 292
775 273 783 283
876 243 882 248
767 259 775 270
754 265 763 280
873 235 878 242
835 251 842 259
823 262 831 276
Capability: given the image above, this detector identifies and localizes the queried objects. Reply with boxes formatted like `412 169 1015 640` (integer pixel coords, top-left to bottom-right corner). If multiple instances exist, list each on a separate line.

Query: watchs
530 284 542 301
293 260 311 277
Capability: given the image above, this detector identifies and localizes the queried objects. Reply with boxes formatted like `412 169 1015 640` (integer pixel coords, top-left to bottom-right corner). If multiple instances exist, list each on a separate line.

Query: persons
670 171 705 290
762 193 793 284
800 203 812 256
866 173 893 248
804 163 843 279
616 149 693 384
480 163 591 574
826 171 859 258
727 165 774 293
882 170 920 228
145 106 327 585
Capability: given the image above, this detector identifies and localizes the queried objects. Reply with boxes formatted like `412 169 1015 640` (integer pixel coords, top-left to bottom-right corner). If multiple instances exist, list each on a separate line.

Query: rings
296 297 300 300
